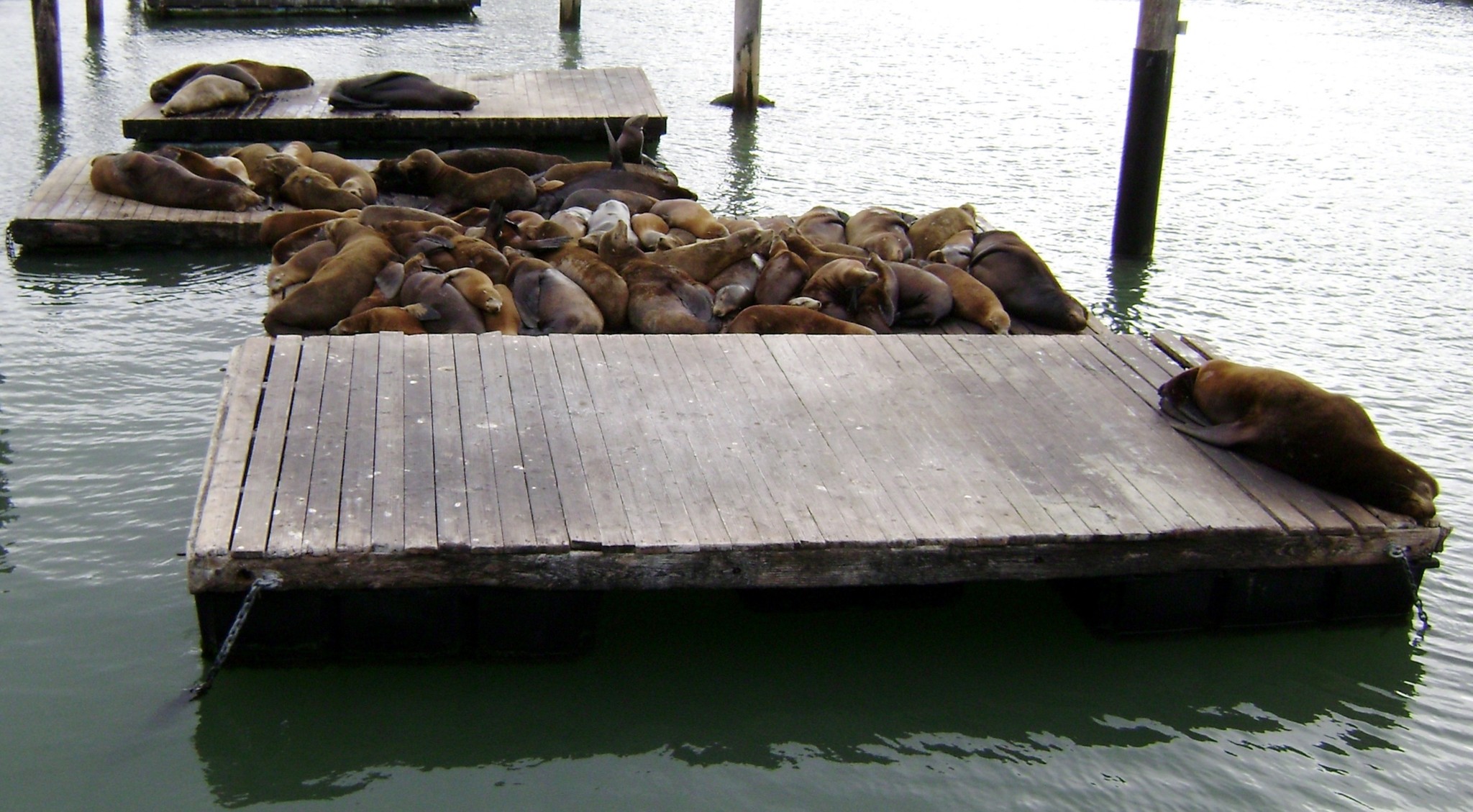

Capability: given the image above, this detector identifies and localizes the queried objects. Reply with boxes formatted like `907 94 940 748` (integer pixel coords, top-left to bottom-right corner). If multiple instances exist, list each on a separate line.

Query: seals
91 58 1092 336
1158 358 1439 520
